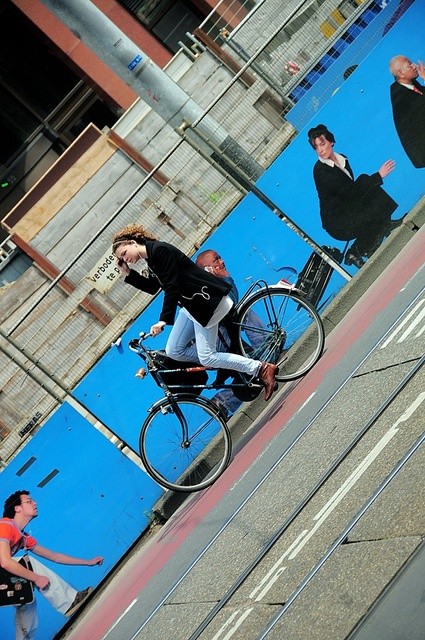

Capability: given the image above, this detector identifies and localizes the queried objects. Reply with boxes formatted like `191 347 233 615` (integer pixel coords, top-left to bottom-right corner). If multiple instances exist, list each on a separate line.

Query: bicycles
128 279 326 493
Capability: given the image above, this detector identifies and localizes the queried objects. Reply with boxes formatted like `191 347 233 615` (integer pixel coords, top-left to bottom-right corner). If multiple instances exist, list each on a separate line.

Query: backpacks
130 341 208 395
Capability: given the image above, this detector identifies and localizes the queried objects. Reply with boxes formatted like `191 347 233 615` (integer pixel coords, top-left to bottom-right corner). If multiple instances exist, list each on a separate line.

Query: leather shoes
379 213 408 238
259 361 279 401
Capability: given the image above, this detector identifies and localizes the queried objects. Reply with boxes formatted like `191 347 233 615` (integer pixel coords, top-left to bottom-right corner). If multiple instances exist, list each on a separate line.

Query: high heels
344 249 365 269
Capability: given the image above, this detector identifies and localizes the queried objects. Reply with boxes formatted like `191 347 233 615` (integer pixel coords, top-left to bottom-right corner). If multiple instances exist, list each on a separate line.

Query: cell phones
204 266 219 273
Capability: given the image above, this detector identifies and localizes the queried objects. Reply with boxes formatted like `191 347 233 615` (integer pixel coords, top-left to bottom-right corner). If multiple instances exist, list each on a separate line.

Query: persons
194 248 295 365
1 488 105 640
387 54 425 169
306 123 409 270
111 223 280 402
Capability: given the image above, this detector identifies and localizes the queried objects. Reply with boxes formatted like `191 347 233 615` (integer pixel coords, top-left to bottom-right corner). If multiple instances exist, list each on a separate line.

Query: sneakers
64 587 94 617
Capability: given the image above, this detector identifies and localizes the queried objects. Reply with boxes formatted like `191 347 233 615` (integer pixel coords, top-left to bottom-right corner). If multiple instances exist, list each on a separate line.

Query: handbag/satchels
0 555 34 606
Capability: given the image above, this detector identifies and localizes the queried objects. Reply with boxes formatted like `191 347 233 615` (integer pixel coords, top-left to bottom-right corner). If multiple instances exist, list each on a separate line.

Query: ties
413 85 423 94
